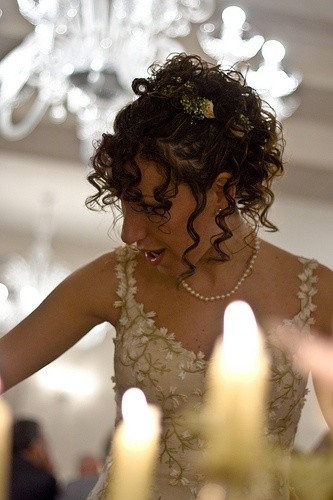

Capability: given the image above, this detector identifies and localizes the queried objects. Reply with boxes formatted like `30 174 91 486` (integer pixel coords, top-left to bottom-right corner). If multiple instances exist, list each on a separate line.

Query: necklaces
177 222 259 301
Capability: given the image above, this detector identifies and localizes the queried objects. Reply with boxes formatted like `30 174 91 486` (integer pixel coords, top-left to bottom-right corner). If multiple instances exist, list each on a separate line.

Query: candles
198 298 272 479
109 385 164 500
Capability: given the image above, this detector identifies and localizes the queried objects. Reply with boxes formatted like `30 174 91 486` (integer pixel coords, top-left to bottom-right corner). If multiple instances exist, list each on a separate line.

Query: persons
78 457 98 475
0 52 332 500
10 420 62 498
